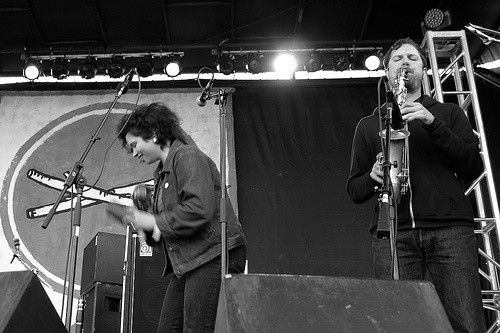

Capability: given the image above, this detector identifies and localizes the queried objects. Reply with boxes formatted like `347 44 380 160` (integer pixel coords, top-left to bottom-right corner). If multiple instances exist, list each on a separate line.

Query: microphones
116 69 136 94
384 76 404 131
13 238 20 249
196 79 213 107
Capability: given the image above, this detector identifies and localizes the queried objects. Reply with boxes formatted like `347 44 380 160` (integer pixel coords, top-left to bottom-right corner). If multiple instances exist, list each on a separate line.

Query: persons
114 103 248 333
347 38 488 333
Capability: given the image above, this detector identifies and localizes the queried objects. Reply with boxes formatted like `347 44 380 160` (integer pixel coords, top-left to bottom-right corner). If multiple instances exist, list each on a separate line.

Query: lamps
363 53 381 71
331 52 350 71
162 54 185 77
135 54 157 76
49 58 71 79
215 54 236 76
242 53 264 75
420 7 454 35
21 55 46 83
106 55 129 77
303 51 325 73
77 55 100 79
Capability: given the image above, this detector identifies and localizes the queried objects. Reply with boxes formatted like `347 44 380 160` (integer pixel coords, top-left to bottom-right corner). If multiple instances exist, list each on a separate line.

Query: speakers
80 231 172 333
0 271 69 333
212 272 456 333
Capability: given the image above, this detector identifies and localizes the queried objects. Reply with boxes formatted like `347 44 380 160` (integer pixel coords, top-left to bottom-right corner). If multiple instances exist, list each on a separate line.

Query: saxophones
378 67 410 205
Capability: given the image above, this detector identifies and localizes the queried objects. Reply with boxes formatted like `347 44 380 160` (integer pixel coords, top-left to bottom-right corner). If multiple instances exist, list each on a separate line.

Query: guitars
106 183 163 249
